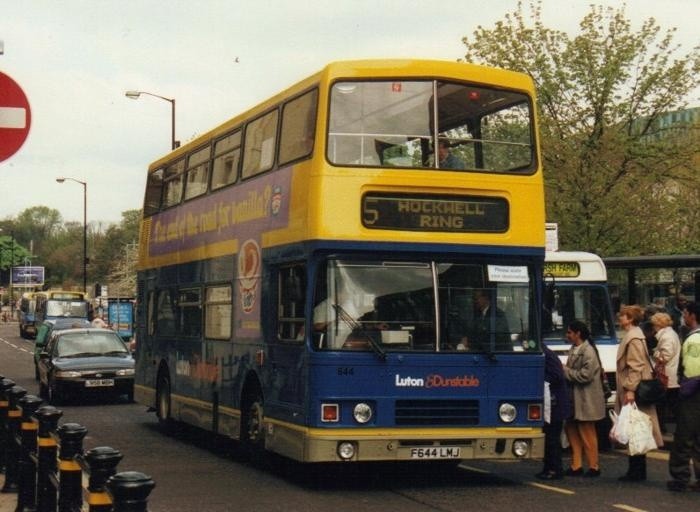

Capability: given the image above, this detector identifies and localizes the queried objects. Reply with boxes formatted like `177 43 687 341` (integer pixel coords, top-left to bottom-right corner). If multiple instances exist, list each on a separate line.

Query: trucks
486 221 622 426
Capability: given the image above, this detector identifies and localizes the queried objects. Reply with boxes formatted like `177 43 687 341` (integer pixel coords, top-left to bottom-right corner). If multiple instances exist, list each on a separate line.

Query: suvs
33 318 91 381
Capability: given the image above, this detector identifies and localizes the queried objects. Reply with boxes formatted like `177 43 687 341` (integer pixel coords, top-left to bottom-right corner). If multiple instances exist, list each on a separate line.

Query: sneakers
536 468 562 480
584 468 600 477
564 465 583 476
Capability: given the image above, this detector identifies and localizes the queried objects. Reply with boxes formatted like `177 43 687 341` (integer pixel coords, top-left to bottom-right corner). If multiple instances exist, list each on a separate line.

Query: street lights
55 175 91 291
124 89 179 154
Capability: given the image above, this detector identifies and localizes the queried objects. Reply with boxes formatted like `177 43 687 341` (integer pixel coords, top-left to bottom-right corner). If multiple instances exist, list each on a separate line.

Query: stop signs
0 70 32 162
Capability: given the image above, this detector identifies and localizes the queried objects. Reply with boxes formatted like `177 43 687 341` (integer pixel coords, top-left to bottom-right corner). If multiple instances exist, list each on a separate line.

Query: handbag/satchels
652 352 668 385
635 379 665 406
601 368 611 398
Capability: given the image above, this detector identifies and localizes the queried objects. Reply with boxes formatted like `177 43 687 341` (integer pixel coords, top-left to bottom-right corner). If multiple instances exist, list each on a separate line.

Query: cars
36 331 135 404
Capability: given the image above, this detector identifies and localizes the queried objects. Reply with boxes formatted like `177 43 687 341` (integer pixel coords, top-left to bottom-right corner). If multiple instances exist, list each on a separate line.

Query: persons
613 304 661 483
667 300 700 492
87 302 112 330
640 293 691 438
429 134 464 170
517 332 569 479
561 322 606 477
310 279 513 354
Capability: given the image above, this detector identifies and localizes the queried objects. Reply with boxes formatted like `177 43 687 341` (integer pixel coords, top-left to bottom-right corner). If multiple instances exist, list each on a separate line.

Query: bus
130 59 551 475
18 292 38 338
34 290 93 322
